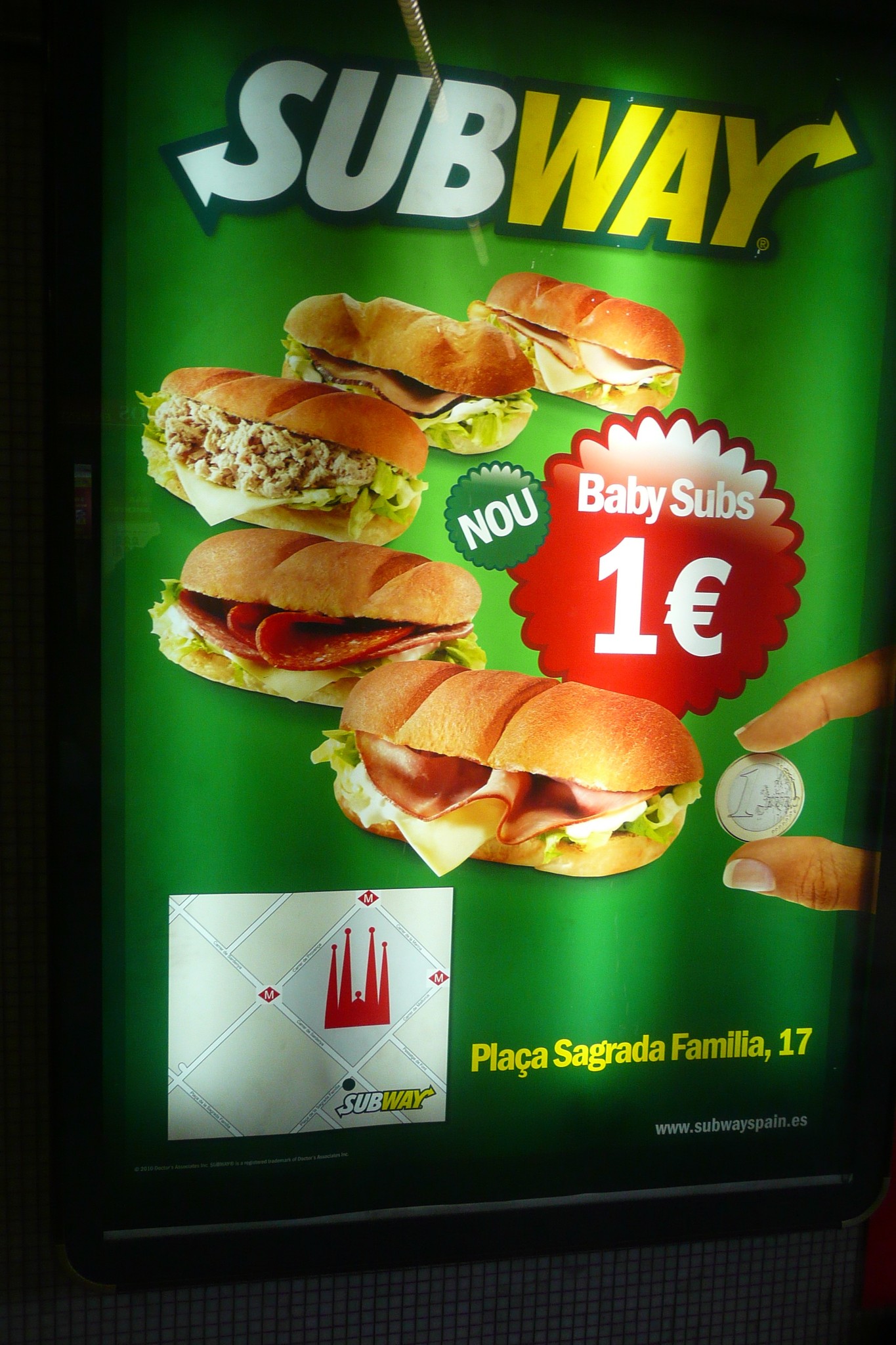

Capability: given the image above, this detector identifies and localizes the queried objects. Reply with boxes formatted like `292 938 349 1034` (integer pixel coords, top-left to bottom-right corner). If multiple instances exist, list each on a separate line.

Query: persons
723 647 895 911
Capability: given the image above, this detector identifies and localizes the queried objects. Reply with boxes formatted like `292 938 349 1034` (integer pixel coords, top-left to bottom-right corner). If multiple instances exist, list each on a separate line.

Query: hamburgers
135 364 434 547
281 287 537 458
144 524 484 714
297 655 702 893
462 270 687 432
